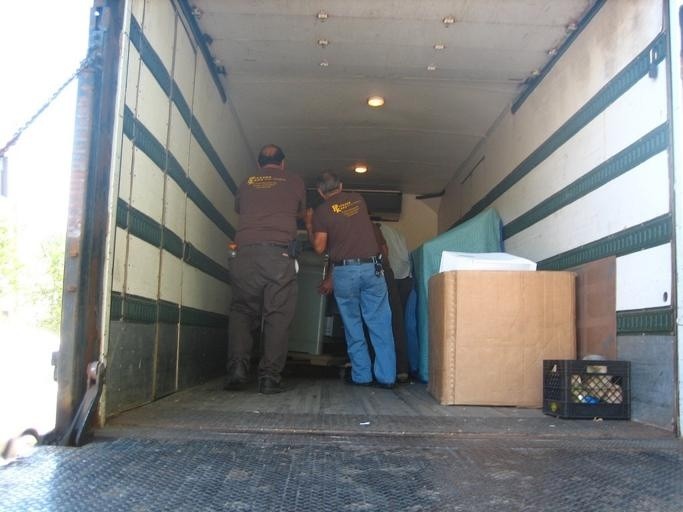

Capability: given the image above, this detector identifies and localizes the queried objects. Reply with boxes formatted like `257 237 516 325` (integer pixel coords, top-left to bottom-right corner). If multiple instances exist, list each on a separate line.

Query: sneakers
259 376 296 395
221 360 253 391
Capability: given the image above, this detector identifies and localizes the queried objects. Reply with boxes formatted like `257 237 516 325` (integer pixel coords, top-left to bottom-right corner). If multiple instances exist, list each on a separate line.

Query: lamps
367 96 385 107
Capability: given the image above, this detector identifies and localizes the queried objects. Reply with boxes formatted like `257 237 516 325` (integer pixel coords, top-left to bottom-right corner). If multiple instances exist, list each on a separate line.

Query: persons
373 216 413 306
222 143 307 396
307 167 397 390
317 222 409 386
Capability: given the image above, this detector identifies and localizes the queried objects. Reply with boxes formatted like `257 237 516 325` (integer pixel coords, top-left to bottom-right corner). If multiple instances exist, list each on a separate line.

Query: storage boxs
542 359 631 420
427 270 579 409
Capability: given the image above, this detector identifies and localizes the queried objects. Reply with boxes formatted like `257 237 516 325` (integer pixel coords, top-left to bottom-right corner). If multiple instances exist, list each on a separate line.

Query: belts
238 241 291 251
332 256 379 267
